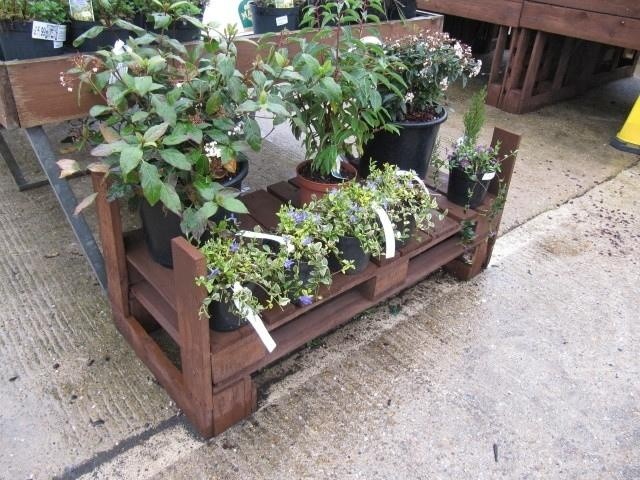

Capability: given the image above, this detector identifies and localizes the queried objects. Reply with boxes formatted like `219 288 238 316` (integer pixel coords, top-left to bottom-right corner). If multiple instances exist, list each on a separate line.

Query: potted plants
0 1 497 332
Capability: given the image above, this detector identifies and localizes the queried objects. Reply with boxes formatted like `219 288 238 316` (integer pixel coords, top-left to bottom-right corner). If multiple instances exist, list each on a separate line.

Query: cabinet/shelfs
90 127 520 436
416 1 640 113
